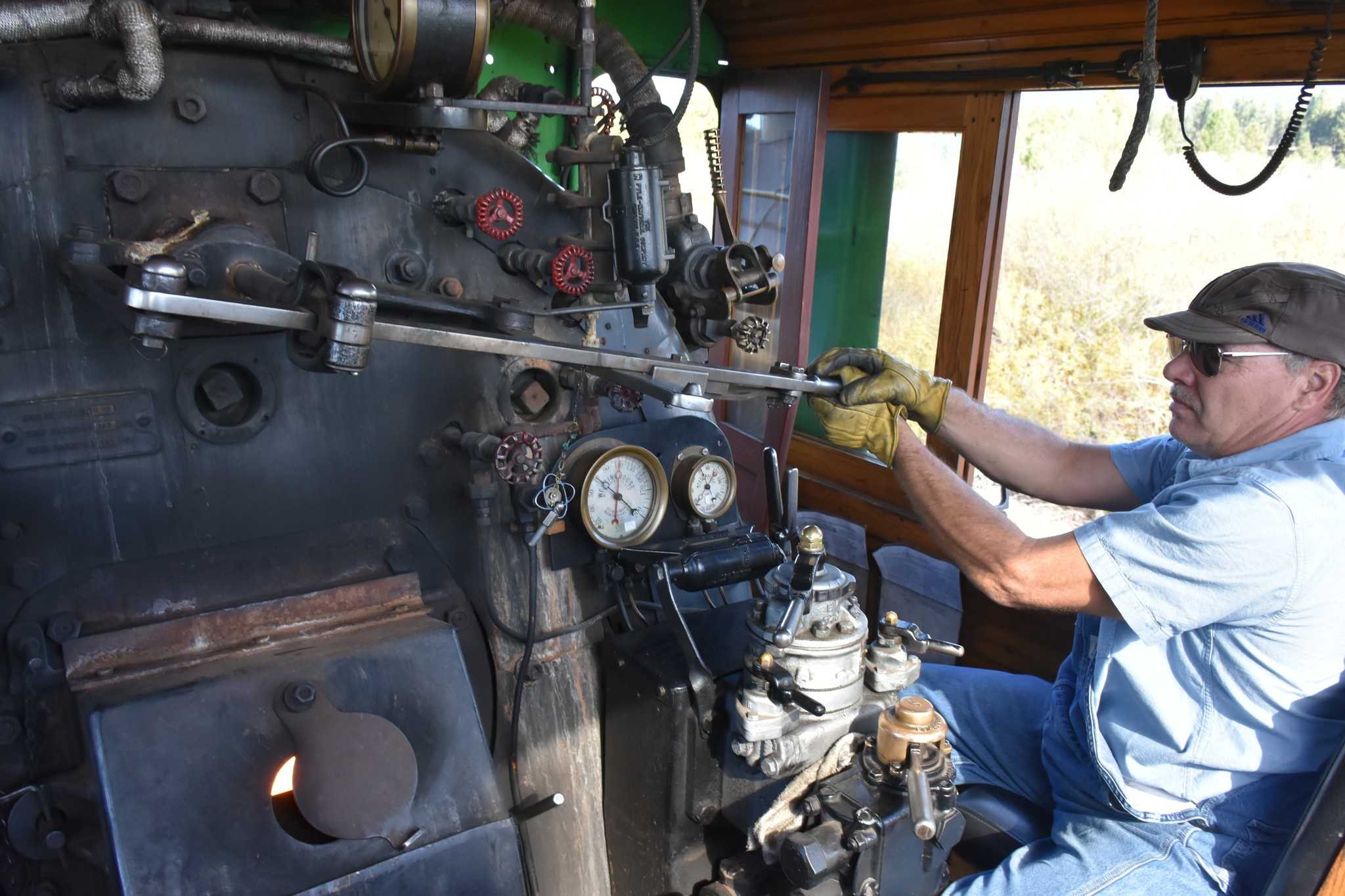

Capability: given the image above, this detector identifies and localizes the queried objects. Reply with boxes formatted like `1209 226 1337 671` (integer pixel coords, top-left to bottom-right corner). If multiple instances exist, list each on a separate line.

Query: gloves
804 347 952 434
807 365 909 470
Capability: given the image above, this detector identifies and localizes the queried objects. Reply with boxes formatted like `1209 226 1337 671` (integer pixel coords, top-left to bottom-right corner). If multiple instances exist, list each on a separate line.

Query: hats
1143 262 1344 368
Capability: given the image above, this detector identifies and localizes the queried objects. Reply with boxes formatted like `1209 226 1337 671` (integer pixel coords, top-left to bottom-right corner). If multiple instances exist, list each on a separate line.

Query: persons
806 262 1345 896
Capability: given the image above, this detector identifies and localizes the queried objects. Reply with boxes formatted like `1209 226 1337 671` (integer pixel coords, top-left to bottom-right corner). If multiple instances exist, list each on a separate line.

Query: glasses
1166 331 1290 377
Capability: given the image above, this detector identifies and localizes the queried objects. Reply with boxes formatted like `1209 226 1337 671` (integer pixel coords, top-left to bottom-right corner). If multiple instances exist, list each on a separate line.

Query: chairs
954 733 1345 896
